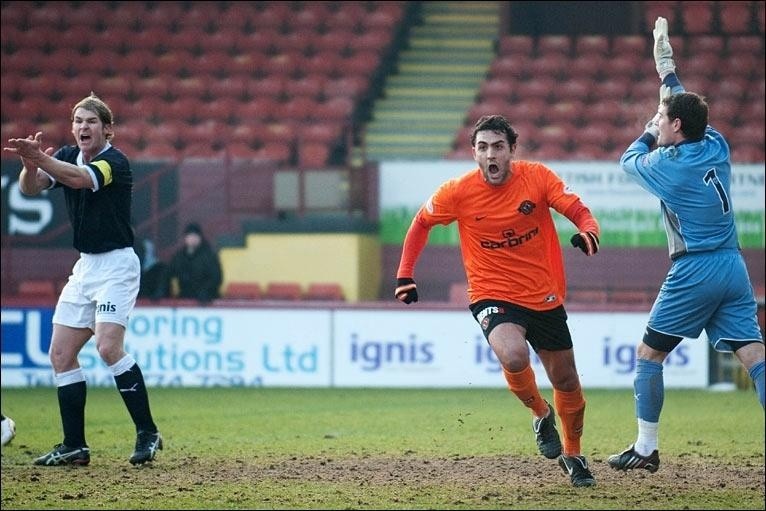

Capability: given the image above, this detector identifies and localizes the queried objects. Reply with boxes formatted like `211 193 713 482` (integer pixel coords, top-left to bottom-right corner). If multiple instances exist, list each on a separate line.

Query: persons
3 91 163 467
605 16 766 473
131 220 223 306
394 113 601 487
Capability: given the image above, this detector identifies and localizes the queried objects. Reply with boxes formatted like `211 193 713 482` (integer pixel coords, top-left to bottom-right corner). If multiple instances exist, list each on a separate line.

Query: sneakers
608 444 660 473
559 455 596 487
532 399 561 459
1 415 16 446
35 443 90 466
129 431 163 466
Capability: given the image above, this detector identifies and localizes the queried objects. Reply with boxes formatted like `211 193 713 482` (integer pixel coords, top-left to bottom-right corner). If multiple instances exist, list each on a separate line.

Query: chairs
446 1 765 165
0 2 425 165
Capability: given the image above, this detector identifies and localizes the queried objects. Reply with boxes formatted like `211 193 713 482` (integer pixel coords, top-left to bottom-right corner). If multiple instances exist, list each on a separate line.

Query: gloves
570 231 600 256
394 278 418 305
645 84 670 142
653 17 676 78
